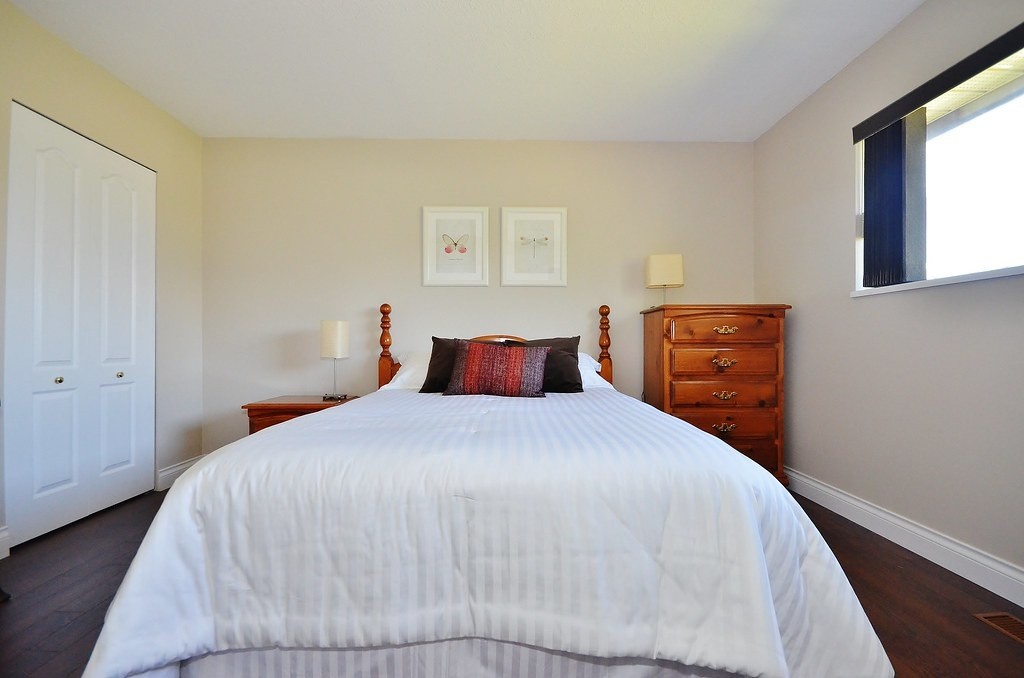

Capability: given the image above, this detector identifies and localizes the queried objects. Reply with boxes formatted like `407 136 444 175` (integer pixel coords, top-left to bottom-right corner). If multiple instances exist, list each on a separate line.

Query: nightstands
639 302 793 486
240 395 360 436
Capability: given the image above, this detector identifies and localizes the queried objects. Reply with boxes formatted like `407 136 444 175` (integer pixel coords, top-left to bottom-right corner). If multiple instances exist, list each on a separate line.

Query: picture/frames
422 206 489 286
500 206 568 287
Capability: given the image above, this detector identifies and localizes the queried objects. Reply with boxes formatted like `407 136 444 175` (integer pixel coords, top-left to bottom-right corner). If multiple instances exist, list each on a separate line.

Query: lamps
320 320 352 401
644 252 684 306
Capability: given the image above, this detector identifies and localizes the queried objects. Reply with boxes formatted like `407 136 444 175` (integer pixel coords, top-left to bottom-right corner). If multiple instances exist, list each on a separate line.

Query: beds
84 369 898 678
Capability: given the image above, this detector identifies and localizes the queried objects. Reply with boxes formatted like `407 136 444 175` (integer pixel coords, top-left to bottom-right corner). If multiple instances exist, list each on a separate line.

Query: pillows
578 353 602 371
541 351 585 393
505 335 580 364
417 336 454 394
380 351 432 390
443 338 552 397
